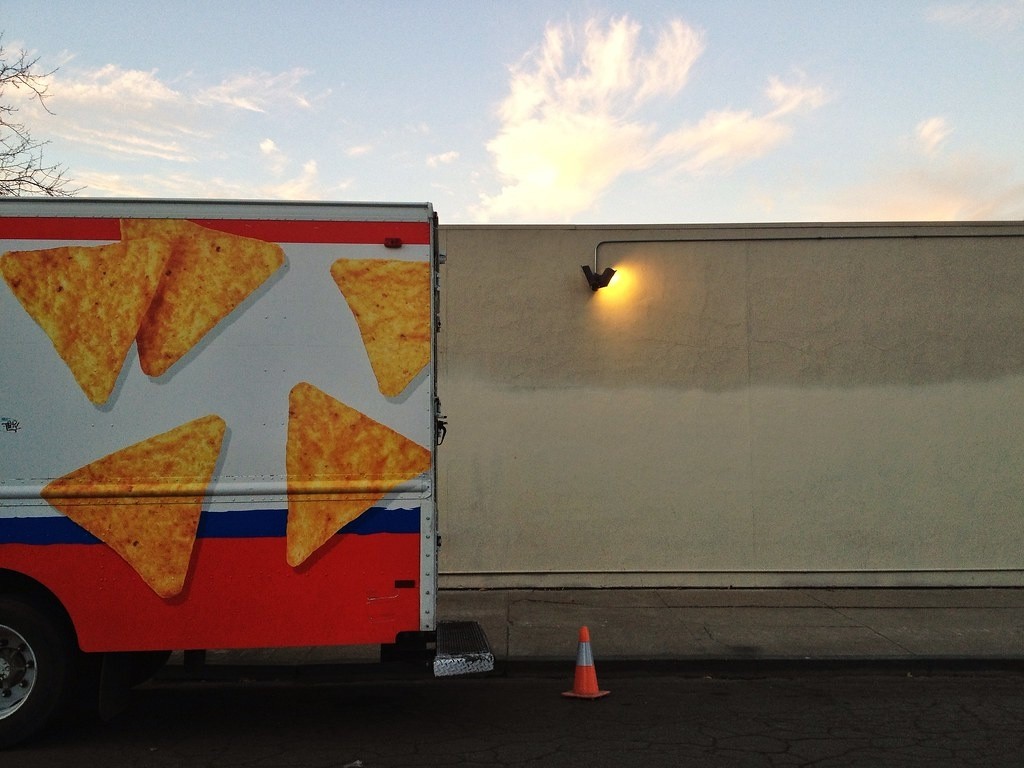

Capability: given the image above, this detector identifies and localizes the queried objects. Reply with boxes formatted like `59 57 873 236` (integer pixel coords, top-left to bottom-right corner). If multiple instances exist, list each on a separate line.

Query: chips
1 219 431 600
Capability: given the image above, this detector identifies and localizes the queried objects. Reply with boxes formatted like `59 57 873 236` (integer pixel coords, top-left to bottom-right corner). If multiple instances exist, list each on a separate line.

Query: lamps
578 264 617 290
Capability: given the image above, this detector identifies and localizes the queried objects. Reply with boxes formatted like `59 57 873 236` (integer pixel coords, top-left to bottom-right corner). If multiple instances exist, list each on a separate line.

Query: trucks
0 195 445 736
